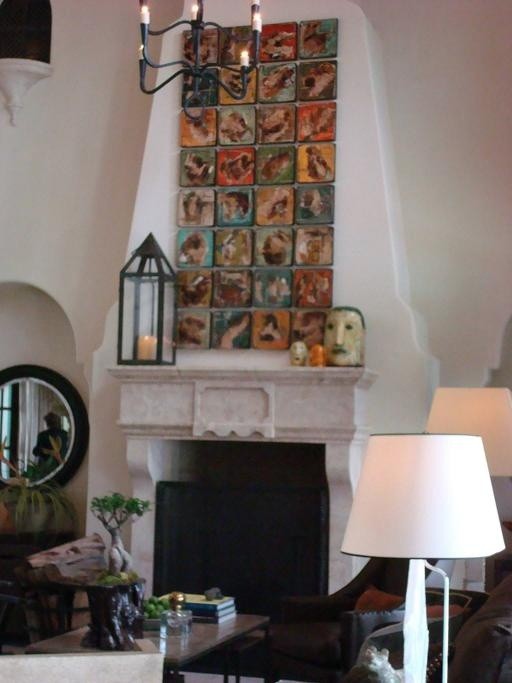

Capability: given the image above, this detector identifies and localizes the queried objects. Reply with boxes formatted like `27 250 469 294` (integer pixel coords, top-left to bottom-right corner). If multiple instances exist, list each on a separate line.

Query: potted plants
79 492 152 650
2 478 79 534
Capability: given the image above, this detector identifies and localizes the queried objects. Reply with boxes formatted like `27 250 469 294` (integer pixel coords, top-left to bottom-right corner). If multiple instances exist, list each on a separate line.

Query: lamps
341 434 507 683
137 1 259 120
426 386 512 478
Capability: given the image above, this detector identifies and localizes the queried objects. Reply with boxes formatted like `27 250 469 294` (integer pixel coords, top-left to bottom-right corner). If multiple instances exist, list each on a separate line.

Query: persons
32 412 68 465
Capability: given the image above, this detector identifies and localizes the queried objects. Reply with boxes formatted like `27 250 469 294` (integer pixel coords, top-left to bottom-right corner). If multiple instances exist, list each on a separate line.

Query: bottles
159 591 193 639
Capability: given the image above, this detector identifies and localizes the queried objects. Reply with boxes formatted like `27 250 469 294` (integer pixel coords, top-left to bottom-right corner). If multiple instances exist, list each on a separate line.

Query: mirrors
0 364 90 492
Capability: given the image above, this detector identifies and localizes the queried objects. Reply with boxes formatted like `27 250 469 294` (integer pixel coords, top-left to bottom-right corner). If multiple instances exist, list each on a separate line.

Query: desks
25 614 271 683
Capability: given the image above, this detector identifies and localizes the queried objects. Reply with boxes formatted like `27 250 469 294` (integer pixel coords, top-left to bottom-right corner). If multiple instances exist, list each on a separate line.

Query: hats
43 412 59 423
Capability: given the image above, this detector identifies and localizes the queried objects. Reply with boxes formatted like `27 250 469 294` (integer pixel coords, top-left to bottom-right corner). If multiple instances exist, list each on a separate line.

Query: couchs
343 573 512 683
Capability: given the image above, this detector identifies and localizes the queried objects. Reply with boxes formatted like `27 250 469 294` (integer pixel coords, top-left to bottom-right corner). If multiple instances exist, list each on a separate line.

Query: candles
136 336 157 360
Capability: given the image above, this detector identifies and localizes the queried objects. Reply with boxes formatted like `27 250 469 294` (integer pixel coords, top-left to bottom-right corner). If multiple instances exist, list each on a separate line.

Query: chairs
266 558 438 683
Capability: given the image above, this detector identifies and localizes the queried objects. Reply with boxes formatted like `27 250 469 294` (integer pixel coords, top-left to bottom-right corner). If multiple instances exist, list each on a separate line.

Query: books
159 592 239 625
